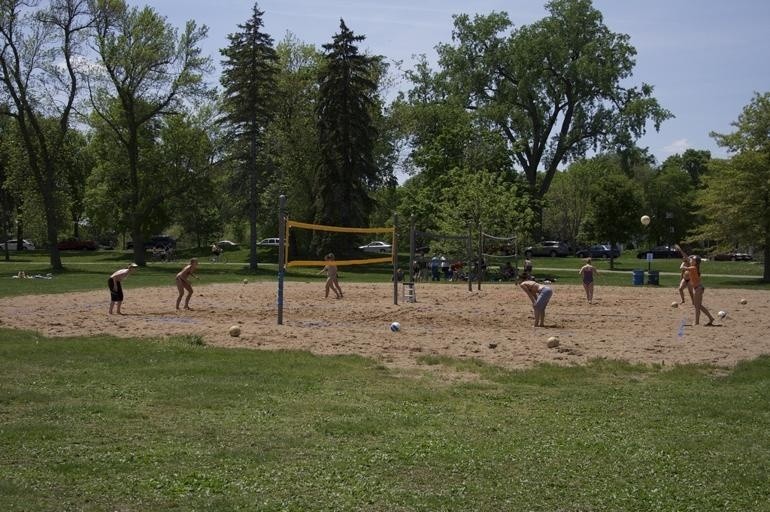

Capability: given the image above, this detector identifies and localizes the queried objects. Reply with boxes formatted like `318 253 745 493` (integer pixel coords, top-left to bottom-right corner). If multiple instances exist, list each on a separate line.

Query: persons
516 272 554 327
440 252 451 281
316 255 339 299
452 258 534 282
428 252 440 281
391 268 405 282
579 258 601 305
675 243 715 326
212 241 222 260
108 263 138 316
175 258 200 310
412 260 421 282
678 256 695 305
329 253 345 298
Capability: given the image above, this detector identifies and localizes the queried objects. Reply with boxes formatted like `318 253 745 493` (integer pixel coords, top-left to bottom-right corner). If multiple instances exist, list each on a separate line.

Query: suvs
523 240 568 258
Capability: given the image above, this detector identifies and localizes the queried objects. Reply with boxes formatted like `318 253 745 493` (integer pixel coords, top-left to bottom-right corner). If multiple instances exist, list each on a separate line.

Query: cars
577 242 621 259
689 255 709 262
127 235 176 251
713 251 753 263
57 237 97 252
360 240 392 254
0 239 35 251
637 245 681 260
218 240 238 247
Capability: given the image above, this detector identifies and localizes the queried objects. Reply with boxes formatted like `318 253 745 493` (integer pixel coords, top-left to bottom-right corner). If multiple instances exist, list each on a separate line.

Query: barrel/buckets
647 270 660 285
632 270 644 285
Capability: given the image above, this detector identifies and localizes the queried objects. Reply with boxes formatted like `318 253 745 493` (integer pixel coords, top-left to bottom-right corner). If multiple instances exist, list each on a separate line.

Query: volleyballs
641 215 650 225
741 299 747 305
547 337 559 348
229 326 240 337
244 279 248 283
718 311 725 317
672 302 678 308
391 322 400 332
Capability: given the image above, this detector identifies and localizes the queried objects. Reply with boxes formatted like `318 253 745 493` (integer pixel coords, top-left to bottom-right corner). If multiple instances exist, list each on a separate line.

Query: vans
257 237 290 248
